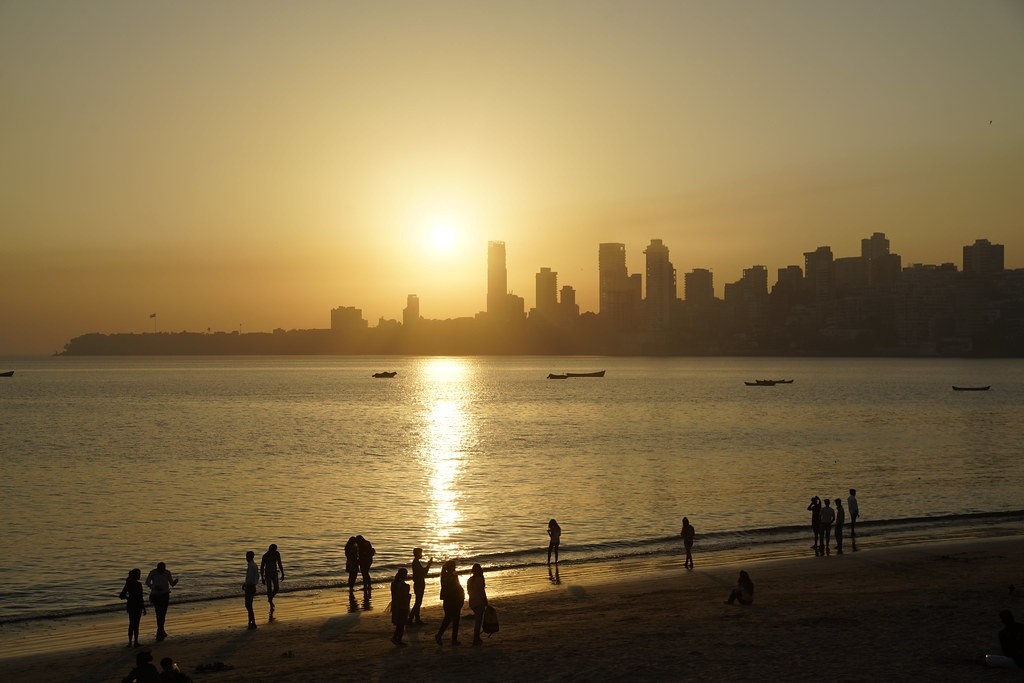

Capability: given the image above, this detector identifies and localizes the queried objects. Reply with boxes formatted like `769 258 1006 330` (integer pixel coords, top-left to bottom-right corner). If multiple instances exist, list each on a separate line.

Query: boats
744 379 793 386
566 370 605 377
372 372 397 378
0 371 14 376
547 373 568 379
952 385 991 391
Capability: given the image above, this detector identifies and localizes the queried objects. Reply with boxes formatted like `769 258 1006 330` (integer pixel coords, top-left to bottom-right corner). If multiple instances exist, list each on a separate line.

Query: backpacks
479 605 499 638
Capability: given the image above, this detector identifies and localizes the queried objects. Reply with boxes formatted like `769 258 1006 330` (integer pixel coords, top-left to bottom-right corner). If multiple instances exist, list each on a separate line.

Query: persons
436 560 464 647
467 564 489 644
391 568 411 645
122 648 192 683
547 519 561 564
724 571 754 604
242 551 259 629
145 562 179 641
119 569 146 647
681 517 694 566
260 544 284 623
807 496 844 551
409 548 432 625
847 489 860 537
345 536 375 591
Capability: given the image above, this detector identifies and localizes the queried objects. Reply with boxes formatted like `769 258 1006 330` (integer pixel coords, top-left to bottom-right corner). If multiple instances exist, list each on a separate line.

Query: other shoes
474 639 483 644
390 637 399 645
426 636 443 646
452 641 461 646
